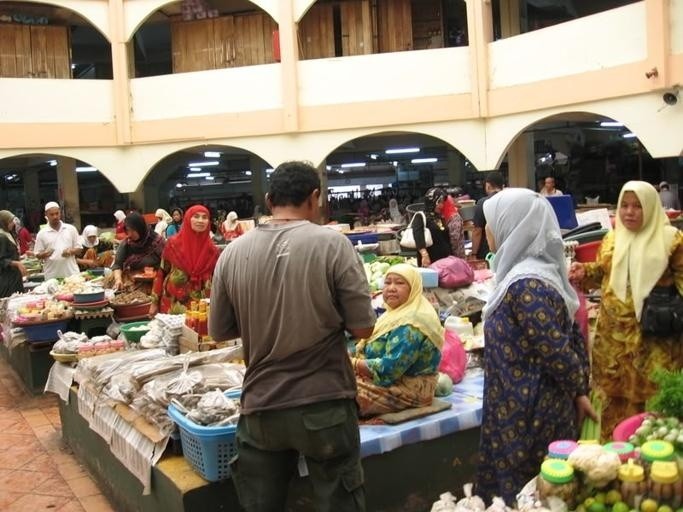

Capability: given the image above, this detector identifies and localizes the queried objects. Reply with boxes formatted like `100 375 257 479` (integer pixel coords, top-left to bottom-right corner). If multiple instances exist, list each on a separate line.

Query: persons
479 187 599 511
210 159 377 511
348 262 445 423
568 182 682 442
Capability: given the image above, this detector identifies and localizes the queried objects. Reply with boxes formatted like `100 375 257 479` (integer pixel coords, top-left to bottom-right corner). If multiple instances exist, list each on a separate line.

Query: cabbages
434 373 452 396
363 262 390 292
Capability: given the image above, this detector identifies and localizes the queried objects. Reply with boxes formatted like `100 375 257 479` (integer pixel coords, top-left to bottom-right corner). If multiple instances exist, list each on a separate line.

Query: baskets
166 385 248 489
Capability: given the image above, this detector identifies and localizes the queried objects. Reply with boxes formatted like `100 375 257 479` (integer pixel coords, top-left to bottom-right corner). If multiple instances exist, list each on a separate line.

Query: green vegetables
580 381 606 440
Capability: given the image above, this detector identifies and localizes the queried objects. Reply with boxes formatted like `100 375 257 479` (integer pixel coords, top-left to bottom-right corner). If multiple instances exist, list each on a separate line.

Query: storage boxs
178 335 229 355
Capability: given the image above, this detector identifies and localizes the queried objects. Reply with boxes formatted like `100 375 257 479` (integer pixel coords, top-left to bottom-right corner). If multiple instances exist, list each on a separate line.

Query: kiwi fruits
109 289 151 304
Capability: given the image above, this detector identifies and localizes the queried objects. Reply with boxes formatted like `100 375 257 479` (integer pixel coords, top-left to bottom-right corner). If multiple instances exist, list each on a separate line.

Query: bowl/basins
25 287 154 362
331 201 475 266
611 409 653 458
563 220 608 264
665 210 682 219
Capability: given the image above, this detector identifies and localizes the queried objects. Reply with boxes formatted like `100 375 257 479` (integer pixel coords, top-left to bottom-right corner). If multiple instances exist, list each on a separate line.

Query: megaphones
663 86 679 105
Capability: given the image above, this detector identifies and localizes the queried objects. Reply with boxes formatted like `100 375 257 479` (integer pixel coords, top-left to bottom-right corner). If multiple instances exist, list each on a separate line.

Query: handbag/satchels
639 285 683 339
398 210 433 250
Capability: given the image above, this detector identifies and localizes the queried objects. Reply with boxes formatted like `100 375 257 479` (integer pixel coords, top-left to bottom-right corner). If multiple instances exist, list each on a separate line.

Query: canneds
537 439 683 511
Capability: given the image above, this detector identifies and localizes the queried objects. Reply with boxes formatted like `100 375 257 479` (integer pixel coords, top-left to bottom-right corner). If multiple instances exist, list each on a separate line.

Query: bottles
184 299 210 340
440 300 459 325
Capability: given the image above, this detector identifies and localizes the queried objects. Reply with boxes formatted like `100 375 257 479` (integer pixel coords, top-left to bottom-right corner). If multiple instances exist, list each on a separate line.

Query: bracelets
351 358 360 374
